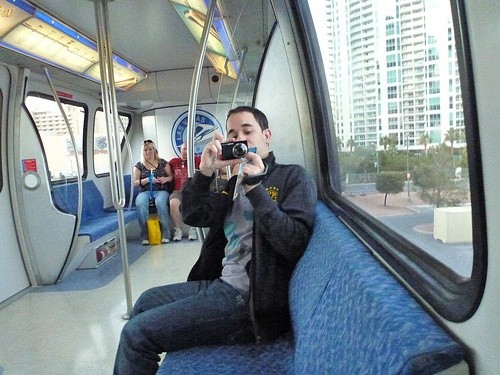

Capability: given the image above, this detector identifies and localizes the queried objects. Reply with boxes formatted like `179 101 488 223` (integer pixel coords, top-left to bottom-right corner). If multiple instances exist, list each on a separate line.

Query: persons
133 140 173 244
168 143 201 242
113 106 317 375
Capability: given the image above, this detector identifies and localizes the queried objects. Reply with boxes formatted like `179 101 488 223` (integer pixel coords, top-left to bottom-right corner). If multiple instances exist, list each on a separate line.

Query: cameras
221 140 248 161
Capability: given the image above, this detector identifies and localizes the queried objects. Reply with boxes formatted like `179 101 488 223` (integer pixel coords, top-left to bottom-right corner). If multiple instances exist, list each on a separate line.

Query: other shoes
161 238 170 243
172 227 183 241
141 240 149 245
188 228 197 240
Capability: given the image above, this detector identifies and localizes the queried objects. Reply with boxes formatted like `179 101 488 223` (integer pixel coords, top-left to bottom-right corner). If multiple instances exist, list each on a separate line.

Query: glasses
143 140 153 143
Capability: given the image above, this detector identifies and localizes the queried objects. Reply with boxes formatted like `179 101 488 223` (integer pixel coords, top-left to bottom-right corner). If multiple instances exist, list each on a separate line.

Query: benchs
152 199 472 375
51 180 138 269
106 175 189 241
